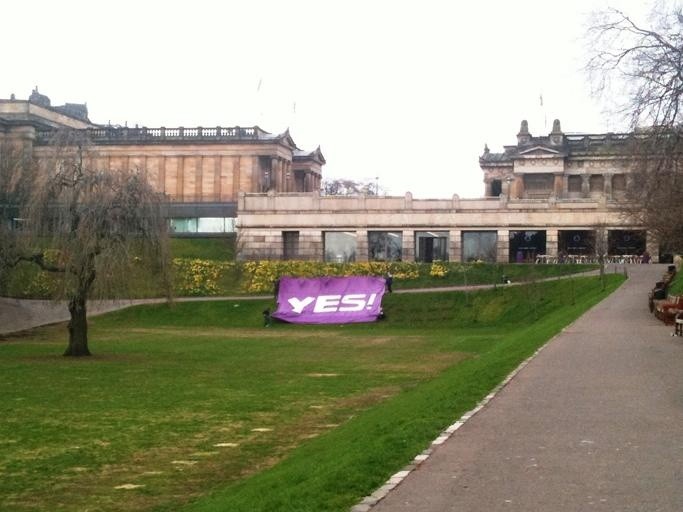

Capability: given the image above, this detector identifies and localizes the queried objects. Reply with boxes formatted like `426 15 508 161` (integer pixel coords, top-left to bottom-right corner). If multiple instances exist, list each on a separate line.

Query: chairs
535 255 644 264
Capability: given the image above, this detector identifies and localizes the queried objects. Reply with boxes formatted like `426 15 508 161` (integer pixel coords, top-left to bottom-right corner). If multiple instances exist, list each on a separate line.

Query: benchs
648 265 683 336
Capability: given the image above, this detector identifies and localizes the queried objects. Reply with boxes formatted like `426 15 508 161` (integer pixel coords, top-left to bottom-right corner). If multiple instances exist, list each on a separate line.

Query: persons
386 272 392 292
263 307 273 328
